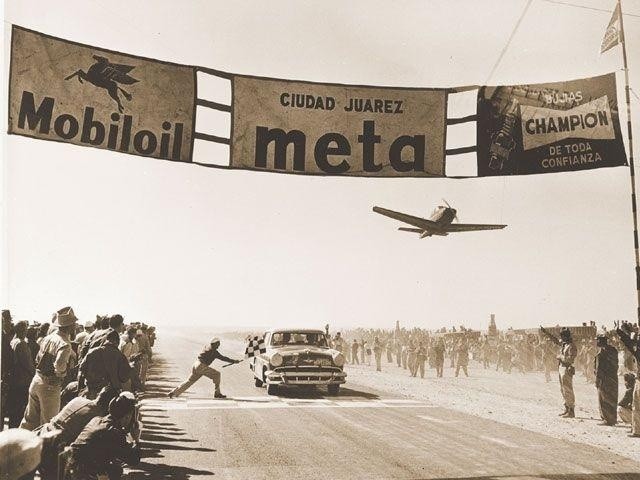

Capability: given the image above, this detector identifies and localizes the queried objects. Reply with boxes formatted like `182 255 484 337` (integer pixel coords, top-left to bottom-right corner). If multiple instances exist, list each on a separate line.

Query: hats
209 337 221 344
50 305 79 327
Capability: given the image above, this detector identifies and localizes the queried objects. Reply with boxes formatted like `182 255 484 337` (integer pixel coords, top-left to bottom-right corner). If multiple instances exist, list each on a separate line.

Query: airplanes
373 199 507 240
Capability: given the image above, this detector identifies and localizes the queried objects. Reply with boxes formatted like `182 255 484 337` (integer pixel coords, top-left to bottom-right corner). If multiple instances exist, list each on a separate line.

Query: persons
3 302 158 480
165 336 240 399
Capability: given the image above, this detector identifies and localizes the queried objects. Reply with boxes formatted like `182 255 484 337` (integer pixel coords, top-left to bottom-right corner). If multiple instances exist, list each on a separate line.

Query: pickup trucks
244 329 347 395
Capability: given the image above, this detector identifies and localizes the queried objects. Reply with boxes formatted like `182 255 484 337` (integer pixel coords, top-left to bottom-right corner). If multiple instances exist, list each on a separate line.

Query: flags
243 331 267 360
595 1 625 57
